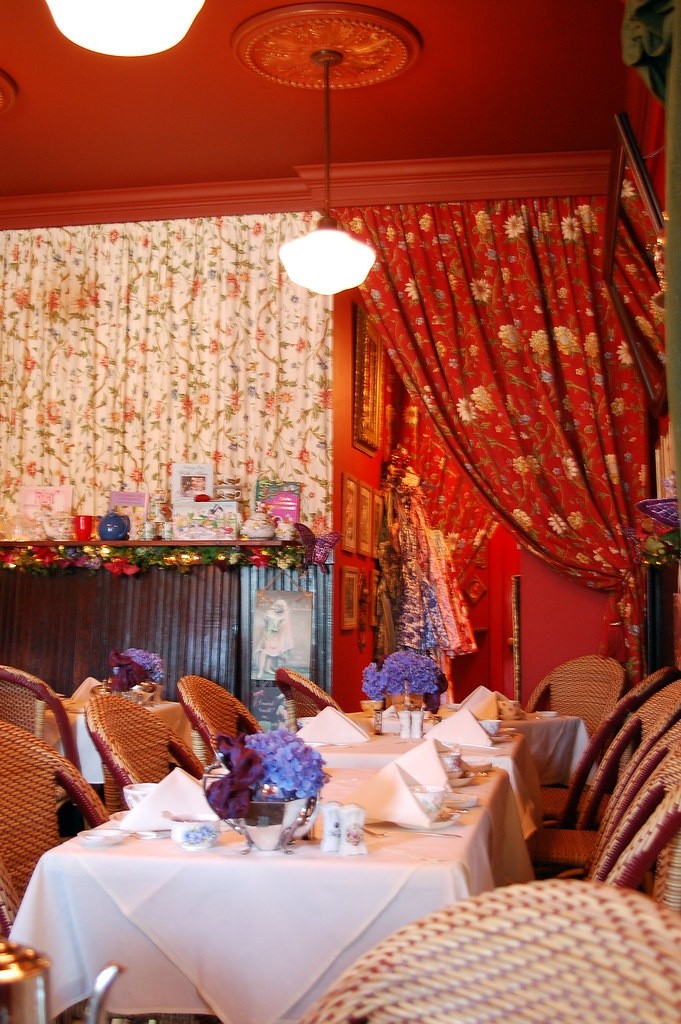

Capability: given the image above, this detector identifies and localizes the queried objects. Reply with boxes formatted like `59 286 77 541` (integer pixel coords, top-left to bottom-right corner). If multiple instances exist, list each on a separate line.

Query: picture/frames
340 566 359 630
372 489 385 559
341 472 359 553
358 481 372 557
368 568 382 626
353 303 384 458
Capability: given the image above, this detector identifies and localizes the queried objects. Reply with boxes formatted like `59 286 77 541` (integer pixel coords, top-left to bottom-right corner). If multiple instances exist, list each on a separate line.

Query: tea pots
240 511 283 540
97 510 129 540
0 511 76 542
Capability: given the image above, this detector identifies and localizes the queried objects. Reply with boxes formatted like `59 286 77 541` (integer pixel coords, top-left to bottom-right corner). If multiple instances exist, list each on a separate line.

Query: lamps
45 0 206 57
278 49 377 296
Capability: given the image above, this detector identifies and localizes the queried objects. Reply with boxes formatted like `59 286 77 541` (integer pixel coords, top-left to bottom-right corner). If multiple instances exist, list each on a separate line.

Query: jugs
0 938 128 1024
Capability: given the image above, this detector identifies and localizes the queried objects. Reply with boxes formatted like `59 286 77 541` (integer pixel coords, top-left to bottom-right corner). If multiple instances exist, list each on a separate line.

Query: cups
411 785 444 817
497 701 519 716
437 750 462 779
275 522 290 540
74 515 92 539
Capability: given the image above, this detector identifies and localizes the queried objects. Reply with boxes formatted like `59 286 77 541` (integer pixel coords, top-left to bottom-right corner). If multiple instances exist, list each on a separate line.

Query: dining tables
345 711 599 790
6 766 535 1024
44 698 194 830
305 732 543 840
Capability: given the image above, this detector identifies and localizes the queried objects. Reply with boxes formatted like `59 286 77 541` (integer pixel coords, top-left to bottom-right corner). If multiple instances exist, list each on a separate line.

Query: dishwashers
143 520 174 541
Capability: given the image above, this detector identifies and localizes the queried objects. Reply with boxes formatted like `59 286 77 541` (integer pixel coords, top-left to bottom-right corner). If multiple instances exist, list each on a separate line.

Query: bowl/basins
500 714 521 719
444 792 478 808
395 813 460 830
536 711 557 717
447 771 474 786
77 828 121 846
171 815 220 850
490 737 505 742
360 700 384 715
108 811 171 840
123 783 159 809
477 720 501 737
466 760 492 771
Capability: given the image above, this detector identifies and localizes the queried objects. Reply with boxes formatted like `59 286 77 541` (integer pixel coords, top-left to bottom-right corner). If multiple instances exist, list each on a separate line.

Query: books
256 480 301 526
172 462 213 505
108 491 150 540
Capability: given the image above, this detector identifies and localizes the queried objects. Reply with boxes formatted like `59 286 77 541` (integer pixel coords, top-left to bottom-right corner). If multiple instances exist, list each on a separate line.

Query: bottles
321 801 364 854
373 713 382 735
397 711 424 738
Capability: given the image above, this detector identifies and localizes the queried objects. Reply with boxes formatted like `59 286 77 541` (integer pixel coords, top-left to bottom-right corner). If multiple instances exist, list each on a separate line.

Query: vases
392 692 424 720
203 752 321 855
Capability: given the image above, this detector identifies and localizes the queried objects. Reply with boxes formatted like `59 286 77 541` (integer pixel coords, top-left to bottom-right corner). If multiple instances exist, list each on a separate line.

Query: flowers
105 647 165 692
361 651 448 701
204 724 334 821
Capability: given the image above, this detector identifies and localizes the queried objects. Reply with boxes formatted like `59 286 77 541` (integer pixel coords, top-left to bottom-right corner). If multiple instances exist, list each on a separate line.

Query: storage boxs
172 497 239 541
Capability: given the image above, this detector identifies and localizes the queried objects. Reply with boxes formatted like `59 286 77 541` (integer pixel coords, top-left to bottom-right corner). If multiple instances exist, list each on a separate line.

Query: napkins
70 677 102 703
119 686 529 832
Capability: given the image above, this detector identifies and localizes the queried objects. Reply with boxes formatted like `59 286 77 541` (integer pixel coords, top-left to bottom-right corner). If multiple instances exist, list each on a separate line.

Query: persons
257 599 295 680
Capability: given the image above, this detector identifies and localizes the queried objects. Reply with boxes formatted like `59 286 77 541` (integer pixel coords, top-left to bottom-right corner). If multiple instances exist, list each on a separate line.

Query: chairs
0 719 110 942
0 664 91 831
276 668 344 718
297 654 681 1024
84 694 208 817
176 675 265 774
